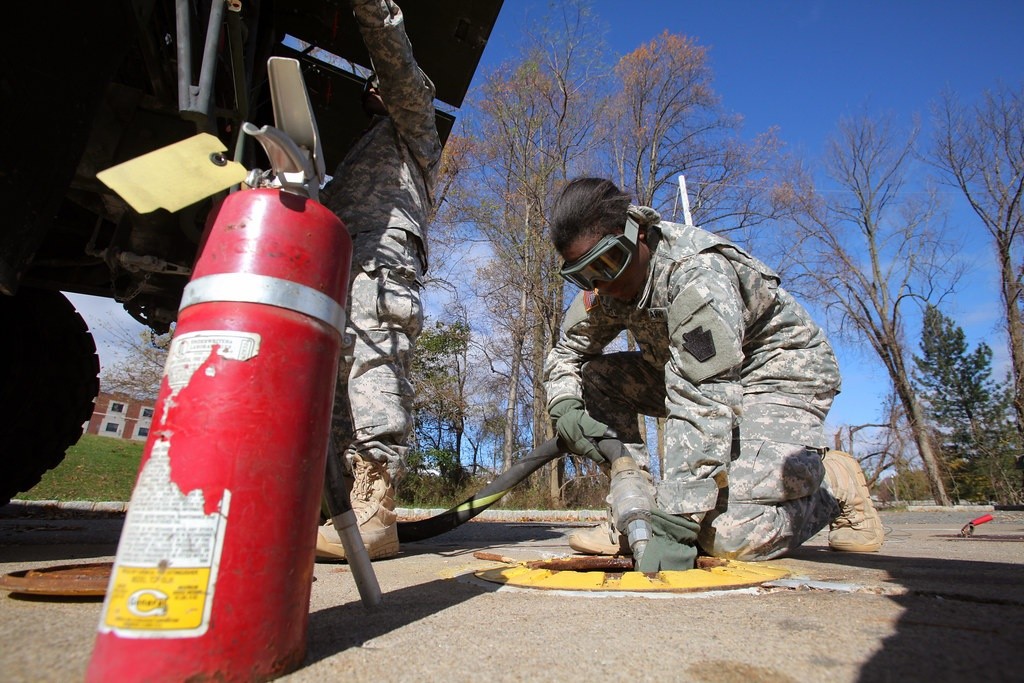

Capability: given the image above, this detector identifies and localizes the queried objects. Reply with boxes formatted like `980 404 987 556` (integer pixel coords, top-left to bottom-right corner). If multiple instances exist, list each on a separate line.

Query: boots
821 449 885 551
569 516 621 554
316 453 399 560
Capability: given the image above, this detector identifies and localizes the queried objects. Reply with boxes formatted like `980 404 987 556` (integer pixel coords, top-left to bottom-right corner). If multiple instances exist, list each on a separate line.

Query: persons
294 0 445 560
532 173 885 575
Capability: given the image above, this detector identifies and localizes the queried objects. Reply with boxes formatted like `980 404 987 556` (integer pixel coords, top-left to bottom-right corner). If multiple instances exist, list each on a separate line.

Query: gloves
548 399 618 468
640 508 700 572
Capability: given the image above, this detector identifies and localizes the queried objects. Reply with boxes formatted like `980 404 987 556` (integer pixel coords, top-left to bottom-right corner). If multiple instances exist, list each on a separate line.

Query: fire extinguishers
82 55 353 683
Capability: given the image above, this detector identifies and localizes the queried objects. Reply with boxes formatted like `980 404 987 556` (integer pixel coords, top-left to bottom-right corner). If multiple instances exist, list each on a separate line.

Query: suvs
0 0 510 510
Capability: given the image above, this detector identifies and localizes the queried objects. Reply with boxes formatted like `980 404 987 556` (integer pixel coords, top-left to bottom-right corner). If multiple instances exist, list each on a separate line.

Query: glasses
559 202 647 290
